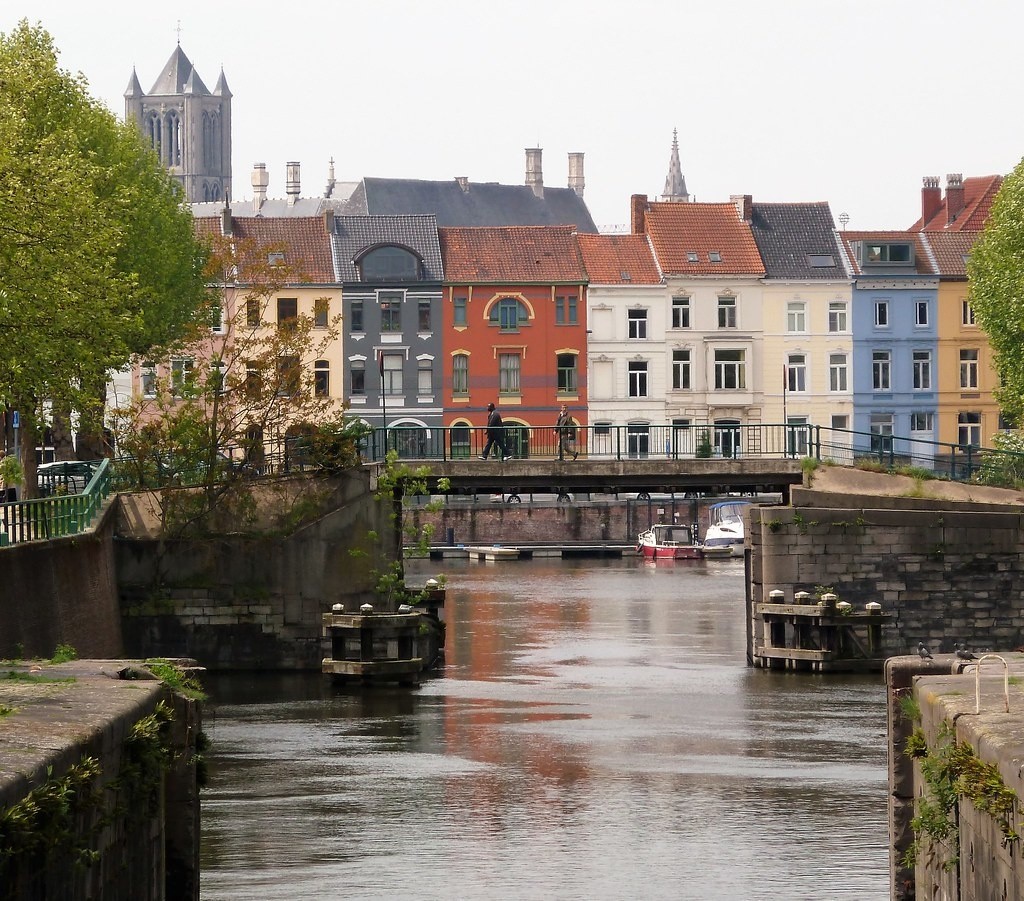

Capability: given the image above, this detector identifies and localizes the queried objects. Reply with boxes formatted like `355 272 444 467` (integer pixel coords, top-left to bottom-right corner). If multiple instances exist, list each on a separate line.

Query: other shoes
555 458 566 461
478 455 487 460
503 455 513 460
573 452 580 462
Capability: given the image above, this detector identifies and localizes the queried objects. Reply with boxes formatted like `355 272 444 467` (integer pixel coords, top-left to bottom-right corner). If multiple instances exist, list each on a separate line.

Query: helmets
486 402 495 407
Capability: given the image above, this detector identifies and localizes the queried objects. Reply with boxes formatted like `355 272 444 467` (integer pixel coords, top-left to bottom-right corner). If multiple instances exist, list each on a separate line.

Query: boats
701 501 750 558
633 522 704 559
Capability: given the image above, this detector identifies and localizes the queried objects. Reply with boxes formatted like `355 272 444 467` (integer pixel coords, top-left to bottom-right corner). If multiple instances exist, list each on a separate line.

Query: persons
553 405 578 462
477 403 512 461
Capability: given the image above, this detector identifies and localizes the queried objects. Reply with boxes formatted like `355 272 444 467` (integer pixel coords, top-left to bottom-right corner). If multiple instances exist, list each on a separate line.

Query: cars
216 450 261 478
35 461 98 488
112 460 184 489
489 493 575 503
626 492 705 499
